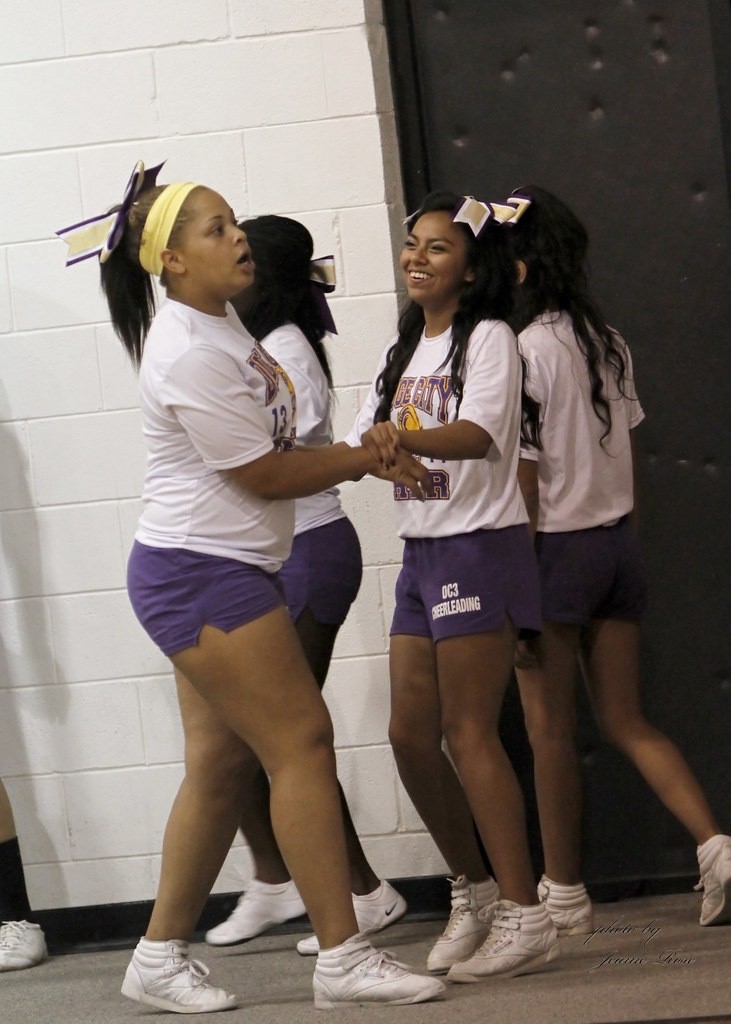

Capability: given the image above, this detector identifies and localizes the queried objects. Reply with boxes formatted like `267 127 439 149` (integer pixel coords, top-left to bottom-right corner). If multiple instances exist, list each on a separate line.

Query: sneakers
120 936 238 1013
693 834 731 925
426 874 500 972
0 920 48 972
537 874 594 937
446 899 562 983
205 878 308 946
312 931 447 1008
297 878 409 955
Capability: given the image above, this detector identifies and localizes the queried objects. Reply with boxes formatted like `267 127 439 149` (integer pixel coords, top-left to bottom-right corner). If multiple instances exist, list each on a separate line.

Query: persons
58 160 446 1013
296 191 562 983
488 183 731 936
0 778 48 971
204 215 406 954
